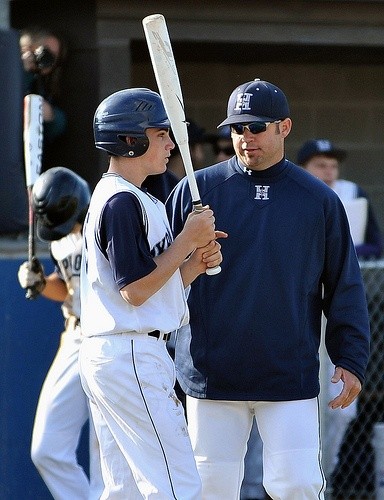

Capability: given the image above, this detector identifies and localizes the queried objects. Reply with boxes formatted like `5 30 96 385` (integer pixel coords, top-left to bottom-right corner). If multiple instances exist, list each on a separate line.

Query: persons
142 115 208 203
17 166 112 500
165 80 384 500
79 88 228 500
18 26 100 179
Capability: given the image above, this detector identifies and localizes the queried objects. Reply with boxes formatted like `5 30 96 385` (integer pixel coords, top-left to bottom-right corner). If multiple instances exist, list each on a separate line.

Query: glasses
213 143 235 155
228 120 282 134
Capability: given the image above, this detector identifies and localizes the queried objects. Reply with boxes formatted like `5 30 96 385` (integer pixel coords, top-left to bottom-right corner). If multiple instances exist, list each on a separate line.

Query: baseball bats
24 94 43 301
142 14 221 275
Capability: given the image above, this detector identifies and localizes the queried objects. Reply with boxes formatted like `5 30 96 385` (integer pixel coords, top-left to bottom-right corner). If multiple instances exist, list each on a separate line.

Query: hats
216 79 290 128
296 139 347 166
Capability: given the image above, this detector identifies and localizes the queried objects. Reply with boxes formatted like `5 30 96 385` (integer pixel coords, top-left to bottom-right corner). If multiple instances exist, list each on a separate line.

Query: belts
148 328 171 341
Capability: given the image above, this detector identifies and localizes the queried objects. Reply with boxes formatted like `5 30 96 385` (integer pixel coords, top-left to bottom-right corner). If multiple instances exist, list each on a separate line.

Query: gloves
17 257 46 297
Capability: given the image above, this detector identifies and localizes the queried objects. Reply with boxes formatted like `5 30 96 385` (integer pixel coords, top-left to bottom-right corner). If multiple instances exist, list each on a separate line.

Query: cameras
32 46 56 71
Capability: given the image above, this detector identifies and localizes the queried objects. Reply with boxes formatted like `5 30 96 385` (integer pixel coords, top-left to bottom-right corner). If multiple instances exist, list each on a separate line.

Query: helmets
93 87 190 158
31 166 90 242
